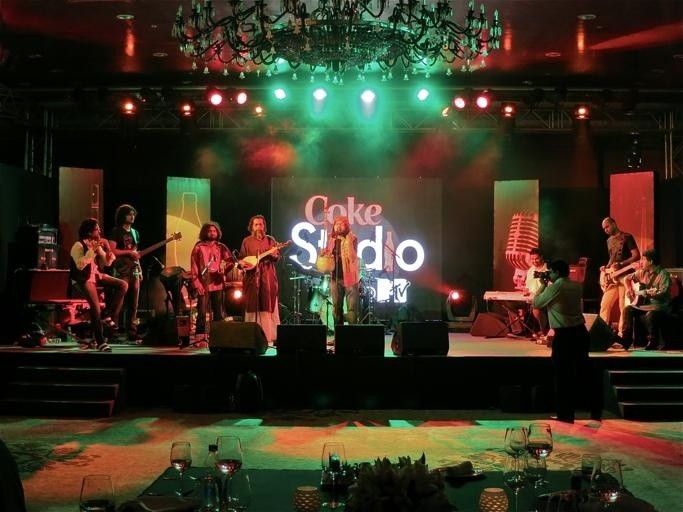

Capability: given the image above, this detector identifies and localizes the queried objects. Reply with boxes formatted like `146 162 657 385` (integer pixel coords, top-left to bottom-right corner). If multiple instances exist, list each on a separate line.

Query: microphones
153 257 165 269
379 266 389 276
256 229 262 240
337 224 342 232
213 254 217 261
504 208 539 290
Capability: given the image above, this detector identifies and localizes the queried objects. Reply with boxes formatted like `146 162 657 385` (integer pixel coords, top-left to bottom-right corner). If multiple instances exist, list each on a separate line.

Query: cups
478 487 509 512
292 485 322 512
77 472 116 512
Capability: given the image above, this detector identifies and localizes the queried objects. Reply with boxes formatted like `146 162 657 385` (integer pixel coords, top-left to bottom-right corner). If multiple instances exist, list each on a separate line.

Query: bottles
66 326 73 343
200 442 221 509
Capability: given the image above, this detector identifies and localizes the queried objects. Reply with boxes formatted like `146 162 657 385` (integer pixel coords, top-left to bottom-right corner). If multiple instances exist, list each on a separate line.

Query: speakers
391 322 450 357
277 323 326 357
209 321 268 356
334 324 385 358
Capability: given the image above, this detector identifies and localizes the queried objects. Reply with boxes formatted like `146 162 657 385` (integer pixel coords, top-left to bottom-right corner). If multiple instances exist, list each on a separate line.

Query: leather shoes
617 334 654 351
551 416 574 424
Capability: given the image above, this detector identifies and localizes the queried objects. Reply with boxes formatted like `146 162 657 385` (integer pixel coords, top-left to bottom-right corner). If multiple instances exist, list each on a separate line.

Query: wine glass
502 454 529 512
215 435 244 503
320 441 348 508
525 423 553 488
503 426 531 472
169 441 193 496
569 452 625 512
226 471 252 512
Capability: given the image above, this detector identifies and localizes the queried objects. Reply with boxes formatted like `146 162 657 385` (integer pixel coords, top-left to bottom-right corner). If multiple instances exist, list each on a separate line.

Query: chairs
632 278 679 349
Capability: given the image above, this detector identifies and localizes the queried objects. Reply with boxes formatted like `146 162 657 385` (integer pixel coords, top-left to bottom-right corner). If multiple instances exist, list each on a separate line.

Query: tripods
279 271 414 337
180 256 214 350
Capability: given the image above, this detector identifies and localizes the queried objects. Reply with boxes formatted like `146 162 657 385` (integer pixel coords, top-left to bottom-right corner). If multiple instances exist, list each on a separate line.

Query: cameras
534 271 549 278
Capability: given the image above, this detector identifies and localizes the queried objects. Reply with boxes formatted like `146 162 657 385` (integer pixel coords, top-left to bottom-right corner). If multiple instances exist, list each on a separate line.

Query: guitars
113 232 182 276
599 260 643 291
624 279 663 306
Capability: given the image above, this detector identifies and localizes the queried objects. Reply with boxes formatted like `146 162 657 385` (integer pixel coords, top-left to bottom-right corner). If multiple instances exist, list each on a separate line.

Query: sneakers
98 343 111 352
102 317 119 329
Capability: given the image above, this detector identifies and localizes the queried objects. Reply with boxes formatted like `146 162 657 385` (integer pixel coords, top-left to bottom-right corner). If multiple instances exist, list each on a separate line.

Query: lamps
446 289 478 322
168 0 504 86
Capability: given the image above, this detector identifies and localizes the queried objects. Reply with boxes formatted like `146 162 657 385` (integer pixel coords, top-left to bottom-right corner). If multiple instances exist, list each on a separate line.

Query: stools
70 277 108 344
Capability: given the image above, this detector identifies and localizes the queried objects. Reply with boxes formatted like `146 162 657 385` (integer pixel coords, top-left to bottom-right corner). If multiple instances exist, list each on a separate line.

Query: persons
321 215 359 344
533 258 604 422
599 216 641 339
107 204 145 345
190 221 236 335
68 218 129 352
238 214 281 347
613 248 672 350
524 248 553 335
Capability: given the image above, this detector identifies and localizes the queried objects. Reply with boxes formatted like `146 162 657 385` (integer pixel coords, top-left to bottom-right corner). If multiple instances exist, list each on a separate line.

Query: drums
316 254 335 274
160 266 187 314
